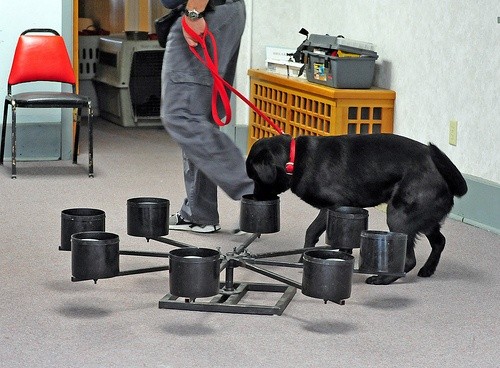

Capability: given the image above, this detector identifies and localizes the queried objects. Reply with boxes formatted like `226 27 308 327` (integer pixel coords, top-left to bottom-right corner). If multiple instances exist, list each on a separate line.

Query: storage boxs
77 35 103 117
92 35 165 128
301 32 380 89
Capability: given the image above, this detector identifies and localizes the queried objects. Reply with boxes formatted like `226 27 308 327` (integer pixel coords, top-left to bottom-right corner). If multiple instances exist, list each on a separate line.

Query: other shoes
82 25 110 36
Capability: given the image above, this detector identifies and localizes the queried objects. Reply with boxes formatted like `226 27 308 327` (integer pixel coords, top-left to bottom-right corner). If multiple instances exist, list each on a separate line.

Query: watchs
185 9 204 19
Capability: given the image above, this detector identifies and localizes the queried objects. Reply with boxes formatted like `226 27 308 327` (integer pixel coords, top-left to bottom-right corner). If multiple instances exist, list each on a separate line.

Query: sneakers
168 212 221 233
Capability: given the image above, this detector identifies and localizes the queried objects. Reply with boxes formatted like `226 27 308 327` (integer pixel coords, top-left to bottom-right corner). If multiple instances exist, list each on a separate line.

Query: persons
160 0 255 233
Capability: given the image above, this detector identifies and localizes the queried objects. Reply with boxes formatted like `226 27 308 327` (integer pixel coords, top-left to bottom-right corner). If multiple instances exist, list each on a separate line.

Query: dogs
244 133 469 287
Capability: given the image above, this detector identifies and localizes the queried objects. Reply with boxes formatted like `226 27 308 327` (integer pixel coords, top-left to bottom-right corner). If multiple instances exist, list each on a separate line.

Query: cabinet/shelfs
246 67 396 157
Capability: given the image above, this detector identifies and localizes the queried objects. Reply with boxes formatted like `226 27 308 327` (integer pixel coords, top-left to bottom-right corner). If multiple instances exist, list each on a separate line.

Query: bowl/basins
168 248 220 298
240 194 280 234
70 232 121 280
125 197 170 236
61 208 106 250
125 31 148 40
360 230 408 273
300 250 356 300
325 206 369 248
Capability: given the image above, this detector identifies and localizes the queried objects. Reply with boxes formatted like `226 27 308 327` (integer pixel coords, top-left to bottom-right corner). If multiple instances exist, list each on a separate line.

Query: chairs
0 27 95 180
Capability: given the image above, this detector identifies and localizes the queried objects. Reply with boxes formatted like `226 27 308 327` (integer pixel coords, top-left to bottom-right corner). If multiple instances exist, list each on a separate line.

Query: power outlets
448 119 459 147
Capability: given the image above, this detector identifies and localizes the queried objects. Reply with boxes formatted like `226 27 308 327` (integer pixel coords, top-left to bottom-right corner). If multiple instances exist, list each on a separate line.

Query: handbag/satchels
154 6 182 49
